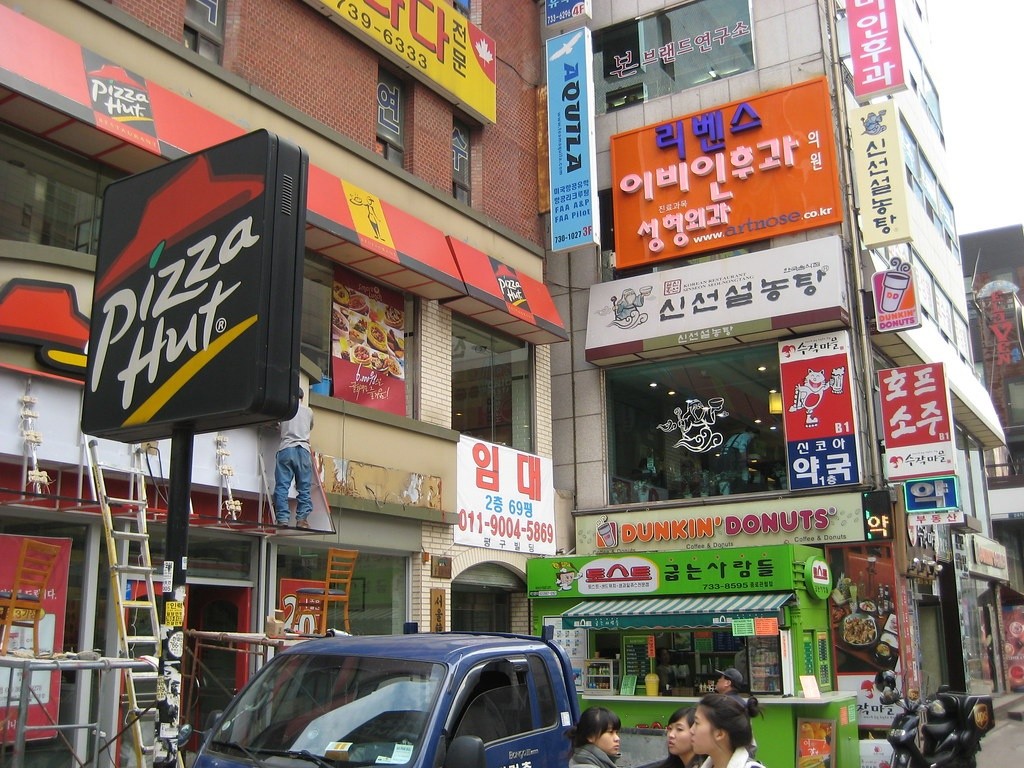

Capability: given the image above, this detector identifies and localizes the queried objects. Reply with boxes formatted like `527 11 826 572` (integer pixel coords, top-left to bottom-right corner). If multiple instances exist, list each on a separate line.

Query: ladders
85 437 187 768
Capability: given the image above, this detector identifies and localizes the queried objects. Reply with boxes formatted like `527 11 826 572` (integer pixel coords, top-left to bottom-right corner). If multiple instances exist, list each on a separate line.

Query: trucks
191 623 679 768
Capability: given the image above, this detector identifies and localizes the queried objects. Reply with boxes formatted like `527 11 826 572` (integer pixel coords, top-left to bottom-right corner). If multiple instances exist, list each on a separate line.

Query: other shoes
277 521 288 526
296 520 311 528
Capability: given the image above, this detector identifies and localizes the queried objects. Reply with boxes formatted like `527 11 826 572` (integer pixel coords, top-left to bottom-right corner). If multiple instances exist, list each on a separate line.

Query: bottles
588 663 610 675
859 571 867 601
588 680 610 689
878 584 890 615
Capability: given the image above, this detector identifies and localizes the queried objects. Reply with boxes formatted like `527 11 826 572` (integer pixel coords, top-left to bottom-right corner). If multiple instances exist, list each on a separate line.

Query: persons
714 669 746 705
690 695 765 768
656 648 676 696
568 706 622 768
274 387 314 528
721 428 759 494
643 706 707 768
633 440 660 502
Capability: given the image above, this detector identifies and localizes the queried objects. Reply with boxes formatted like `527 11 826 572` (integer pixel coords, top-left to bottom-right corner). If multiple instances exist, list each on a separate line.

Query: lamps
900 554 942 585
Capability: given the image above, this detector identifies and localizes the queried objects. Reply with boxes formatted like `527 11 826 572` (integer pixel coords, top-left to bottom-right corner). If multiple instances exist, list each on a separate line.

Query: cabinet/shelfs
582 657 623 696
745 629 785 695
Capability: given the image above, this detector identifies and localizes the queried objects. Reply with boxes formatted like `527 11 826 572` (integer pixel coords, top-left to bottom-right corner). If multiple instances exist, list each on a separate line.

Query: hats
715 668 743 687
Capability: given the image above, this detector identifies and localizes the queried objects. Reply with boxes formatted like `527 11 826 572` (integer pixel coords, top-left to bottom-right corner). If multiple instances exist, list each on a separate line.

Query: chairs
290 548 359 636
0 538 61 659
461 657 532 740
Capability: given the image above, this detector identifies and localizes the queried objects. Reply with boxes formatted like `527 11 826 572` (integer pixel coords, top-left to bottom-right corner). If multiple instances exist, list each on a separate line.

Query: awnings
561 592 794 630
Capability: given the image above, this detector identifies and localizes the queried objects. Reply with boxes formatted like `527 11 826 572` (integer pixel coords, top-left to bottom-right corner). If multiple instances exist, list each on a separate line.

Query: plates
333 307 349 336
885 613 898 636
388 356 404 376
839 613 877 646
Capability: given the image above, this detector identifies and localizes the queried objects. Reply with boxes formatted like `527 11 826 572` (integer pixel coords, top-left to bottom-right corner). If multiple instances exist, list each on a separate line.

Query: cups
594 651 600 659
615 653 621 659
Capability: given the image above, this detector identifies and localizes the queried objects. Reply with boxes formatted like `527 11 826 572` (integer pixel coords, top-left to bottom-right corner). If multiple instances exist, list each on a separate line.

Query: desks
830 598 898 670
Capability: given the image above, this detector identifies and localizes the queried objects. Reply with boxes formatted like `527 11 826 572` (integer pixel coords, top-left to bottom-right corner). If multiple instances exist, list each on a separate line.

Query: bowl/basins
367 321 387 351
350 293 368 312
859 598 878 613
351 345 372 367
874 641 894 666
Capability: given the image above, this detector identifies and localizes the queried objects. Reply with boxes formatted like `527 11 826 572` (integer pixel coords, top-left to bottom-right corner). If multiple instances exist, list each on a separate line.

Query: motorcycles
873 667 994 766
